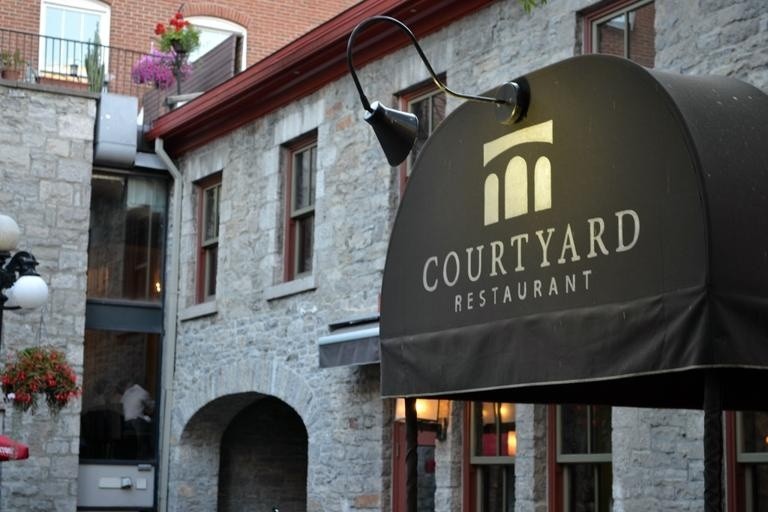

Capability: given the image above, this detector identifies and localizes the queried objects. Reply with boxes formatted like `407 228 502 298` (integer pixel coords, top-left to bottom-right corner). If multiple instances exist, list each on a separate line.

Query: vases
169 37 184 54
156 79 173 89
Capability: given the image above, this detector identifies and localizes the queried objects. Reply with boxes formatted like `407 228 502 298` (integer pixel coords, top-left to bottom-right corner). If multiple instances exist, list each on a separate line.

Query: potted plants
0 48 29 79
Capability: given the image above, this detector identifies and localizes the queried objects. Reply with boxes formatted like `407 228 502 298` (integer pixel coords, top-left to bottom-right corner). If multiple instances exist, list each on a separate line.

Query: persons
119 375 156 458
92 380 114 405
110 378 133 404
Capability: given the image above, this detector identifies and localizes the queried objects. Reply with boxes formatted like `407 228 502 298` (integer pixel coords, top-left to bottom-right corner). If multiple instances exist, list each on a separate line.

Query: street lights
0 213 51 363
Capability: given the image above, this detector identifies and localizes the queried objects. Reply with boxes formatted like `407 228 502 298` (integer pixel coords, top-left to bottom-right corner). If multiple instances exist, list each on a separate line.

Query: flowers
154 10 200 53
131 47 190 87
0 346 83 413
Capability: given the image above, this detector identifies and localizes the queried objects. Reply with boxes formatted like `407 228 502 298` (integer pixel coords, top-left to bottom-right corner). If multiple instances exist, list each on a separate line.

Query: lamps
395 398 448 443
346 16 523 169
